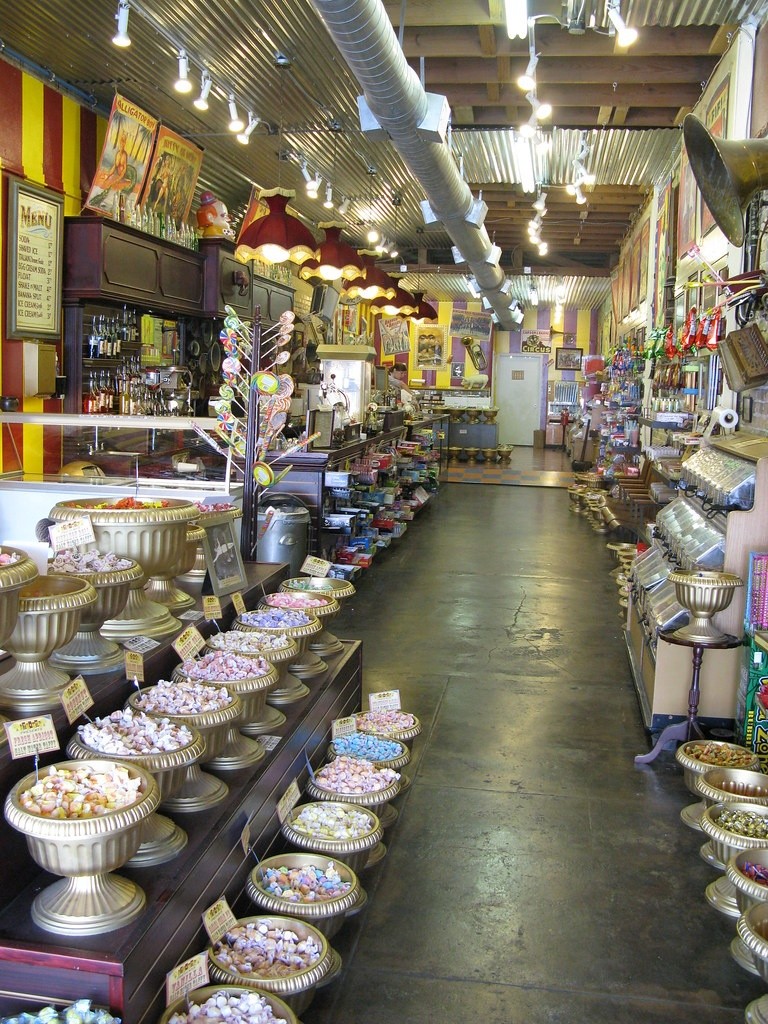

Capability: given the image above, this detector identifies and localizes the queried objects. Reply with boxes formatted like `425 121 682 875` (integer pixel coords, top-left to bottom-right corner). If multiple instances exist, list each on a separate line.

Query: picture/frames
555 348 583 371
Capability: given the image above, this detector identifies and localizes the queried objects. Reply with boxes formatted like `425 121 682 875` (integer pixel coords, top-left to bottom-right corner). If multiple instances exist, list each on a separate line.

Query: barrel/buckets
253 506 310 582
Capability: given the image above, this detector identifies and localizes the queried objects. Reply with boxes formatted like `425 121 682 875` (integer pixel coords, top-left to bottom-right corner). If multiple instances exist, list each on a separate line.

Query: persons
386 362 415 405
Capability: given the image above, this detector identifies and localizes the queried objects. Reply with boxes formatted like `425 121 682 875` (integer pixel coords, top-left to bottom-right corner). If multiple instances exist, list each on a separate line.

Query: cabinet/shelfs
623 433 768 752
62 216 298 478
546 380 605 464
234 413 499 559
1 561 363 1024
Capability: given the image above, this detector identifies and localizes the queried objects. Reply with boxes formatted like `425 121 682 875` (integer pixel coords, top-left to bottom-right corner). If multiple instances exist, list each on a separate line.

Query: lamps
234 59 321 266
524 91 552 119
605 2 637 48
113 0 132 47
376 237 398 258
370 199 419 315
175 49 192 92
341 169 397 300
517 56 540 91
194 72 213 110
299 123 366 282
396 228 439 323
520 114 549 256
306 173 322 199
566 147 596 204
339 195 351 215
237 112 262 144
227 93 244 131
301 161 318 190
322 182 334 209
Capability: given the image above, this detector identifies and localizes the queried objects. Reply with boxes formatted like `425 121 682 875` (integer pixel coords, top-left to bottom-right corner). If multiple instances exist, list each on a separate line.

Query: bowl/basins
0 397 20 412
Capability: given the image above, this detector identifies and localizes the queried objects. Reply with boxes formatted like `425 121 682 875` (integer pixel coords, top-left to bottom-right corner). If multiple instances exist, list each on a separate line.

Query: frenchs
550 325 576 344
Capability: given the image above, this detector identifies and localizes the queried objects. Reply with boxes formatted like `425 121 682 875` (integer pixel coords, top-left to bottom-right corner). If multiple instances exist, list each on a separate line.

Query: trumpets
461 335 487 371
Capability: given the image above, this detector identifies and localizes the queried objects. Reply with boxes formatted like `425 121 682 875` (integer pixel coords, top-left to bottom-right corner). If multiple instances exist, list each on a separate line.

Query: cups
631 431 639 447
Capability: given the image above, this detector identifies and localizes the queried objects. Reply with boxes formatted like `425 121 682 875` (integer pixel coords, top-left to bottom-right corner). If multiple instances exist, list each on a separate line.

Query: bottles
83 305 179 416
